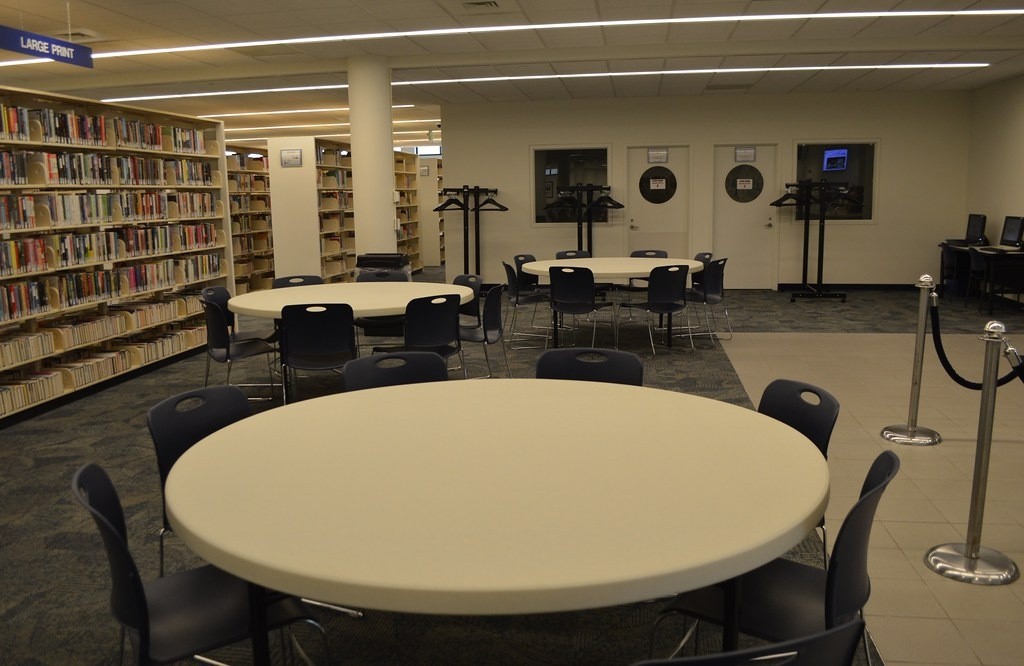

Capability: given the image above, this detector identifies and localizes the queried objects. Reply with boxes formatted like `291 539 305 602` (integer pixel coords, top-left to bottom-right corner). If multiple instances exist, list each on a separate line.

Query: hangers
470 195 509 211
585 185 625 209
830 193 864 209
770 194 812 207
543 199 576 211
433 193 469 211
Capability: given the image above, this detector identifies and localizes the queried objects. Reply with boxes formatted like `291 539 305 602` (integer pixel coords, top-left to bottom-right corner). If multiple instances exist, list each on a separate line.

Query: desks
228 282 474 400
938 244 1024 315
165 378 831 666
521 257 704 349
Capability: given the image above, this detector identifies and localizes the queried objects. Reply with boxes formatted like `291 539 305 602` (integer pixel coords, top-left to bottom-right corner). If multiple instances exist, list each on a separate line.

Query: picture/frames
280 149 302 167
543 181 553 198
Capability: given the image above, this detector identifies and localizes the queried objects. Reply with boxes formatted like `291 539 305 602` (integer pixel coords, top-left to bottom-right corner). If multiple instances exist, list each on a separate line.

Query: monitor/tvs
966 214 986 242
1000 216 1024 247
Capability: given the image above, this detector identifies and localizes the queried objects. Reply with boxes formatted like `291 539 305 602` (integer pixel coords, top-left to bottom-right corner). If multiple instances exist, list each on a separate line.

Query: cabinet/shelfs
0 86 239 418
226 144 273 296
266 137 423 282
419 158 445 267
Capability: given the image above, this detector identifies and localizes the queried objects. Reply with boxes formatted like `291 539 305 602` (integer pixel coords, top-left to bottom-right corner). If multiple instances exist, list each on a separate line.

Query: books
314 144 355 275
0 101 220 418
225 153 274 276
394 190 413 257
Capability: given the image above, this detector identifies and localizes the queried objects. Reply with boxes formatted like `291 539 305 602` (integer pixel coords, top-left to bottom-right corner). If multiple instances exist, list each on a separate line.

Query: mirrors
529 143 613 228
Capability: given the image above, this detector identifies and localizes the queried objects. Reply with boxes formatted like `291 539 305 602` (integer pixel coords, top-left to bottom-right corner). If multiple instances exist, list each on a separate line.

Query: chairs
510 254 575 339
536 348 643 386
353 272 408 358
631 620 865 666
964 247 1003 313
552 251 613 330
272 276 325 385
342 351 448 392
616 266 696 355
201 286 282 386
72 462 331 666
758 378 840 570
372 294 467 378
648 451 900 666
654 253 718 338
679 258 733 346
459 284 513 378
282 304 358 405
941 242 979 302
549 267 616 348
502 261 551 350
619 250 667 322
447 274 483 370
199 299 274 401
146 386 252 579
